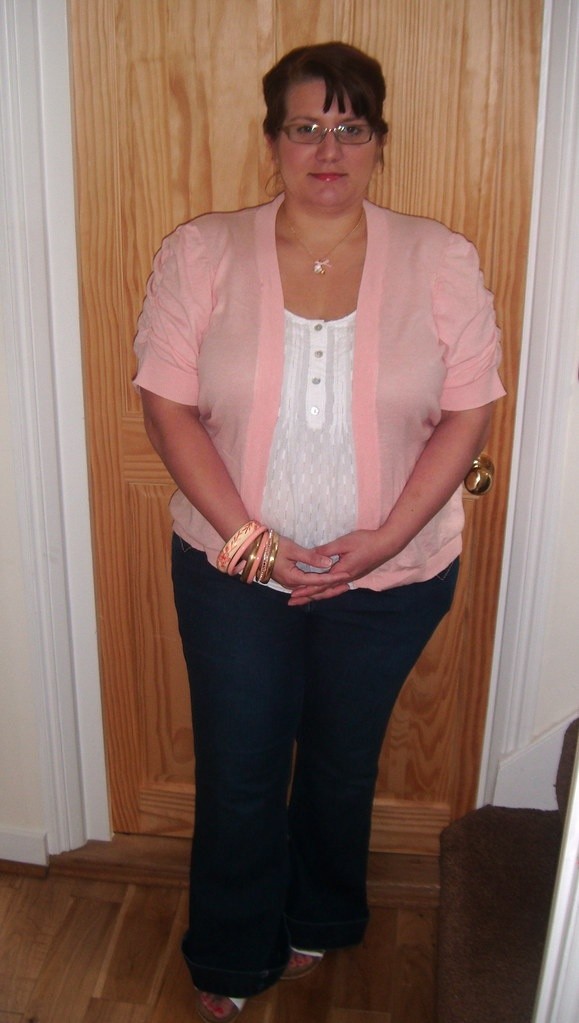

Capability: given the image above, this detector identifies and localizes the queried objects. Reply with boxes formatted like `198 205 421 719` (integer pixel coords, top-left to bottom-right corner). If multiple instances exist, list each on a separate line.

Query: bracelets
216 519 279 585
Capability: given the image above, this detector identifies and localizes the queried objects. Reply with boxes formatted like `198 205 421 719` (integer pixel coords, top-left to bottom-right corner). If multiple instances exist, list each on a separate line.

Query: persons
132 40 507 1023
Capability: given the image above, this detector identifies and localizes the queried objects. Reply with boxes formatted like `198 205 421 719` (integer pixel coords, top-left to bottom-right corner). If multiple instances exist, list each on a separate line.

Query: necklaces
283 208 365 275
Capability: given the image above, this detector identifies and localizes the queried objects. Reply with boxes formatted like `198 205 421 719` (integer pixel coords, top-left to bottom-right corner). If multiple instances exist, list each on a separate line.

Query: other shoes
280 945 325 979
194 989 246 1023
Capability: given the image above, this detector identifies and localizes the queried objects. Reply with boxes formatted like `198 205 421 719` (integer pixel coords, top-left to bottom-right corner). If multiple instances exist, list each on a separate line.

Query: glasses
277 123 375 144
